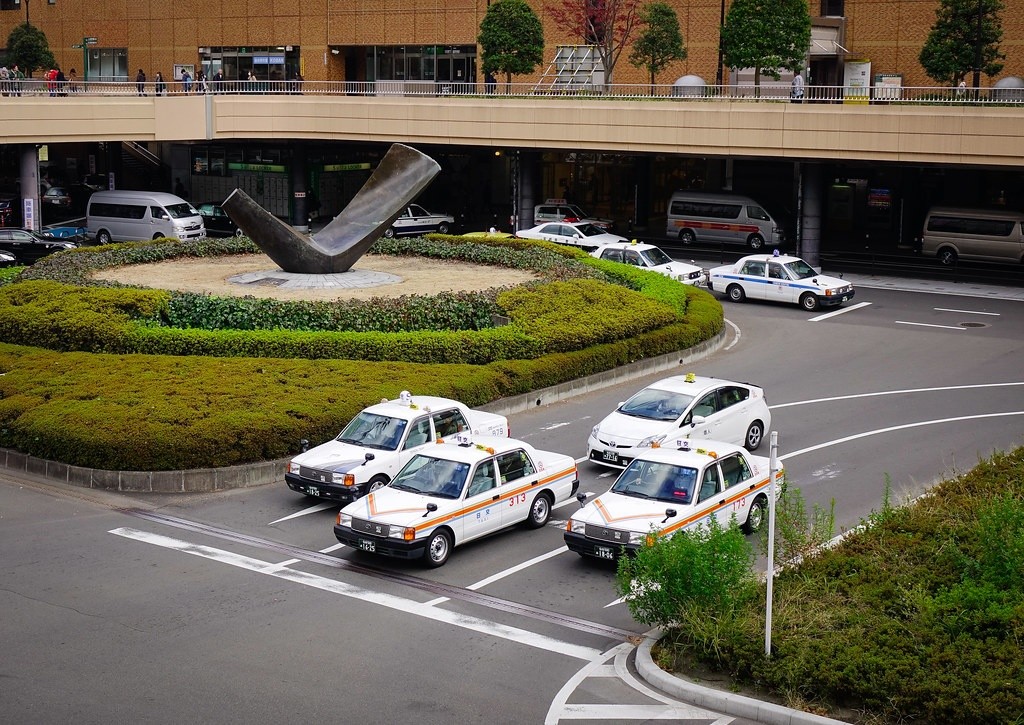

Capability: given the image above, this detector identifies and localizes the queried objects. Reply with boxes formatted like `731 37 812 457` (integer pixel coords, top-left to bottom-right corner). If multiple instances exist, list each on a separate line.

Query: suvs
0 226 82 267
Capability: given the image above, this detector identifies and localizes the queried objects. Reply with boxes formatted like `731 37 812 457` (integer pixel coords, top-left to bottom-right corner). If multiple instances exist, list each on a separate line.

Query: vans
913 206 1024 272
665 191 786 253
85 189 206 245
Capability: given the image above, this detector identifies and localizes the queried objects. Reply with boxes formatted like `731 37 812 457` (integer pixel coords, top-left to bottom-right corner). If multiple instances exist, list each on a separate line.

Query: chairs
687 469 717 506
692 396 716 418
404 420 428 450
469 464 493 498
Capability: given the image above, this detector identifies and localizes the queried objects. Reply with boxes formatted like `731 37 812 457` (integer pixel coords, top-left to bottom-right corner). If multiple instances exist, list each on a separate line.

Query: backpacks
184 75 192 88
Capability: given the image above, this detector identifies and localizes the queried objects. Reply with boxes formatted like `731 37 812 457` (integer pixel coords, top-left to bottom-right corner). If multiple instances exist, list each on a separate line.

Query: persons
791 67 804 103
39 169 53 203
174 178 185 200
0 65 25 97
302 186 322 236
43 67 78 97
957 77 967 100
135 67 304 97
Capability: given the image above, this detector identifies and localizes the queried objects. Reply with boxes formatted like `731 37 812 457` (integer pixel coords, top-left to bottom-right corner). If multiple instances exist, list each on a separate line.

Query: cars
195 201 246 238
586 371 772 484
515 216 630 254
562 437 786 578
283 388 511 506
706 249 855 312
507 198 614 234
332 432 583 568
41 186 73 210
586 238 707 289
0 198 22 228
382 203 455 239
